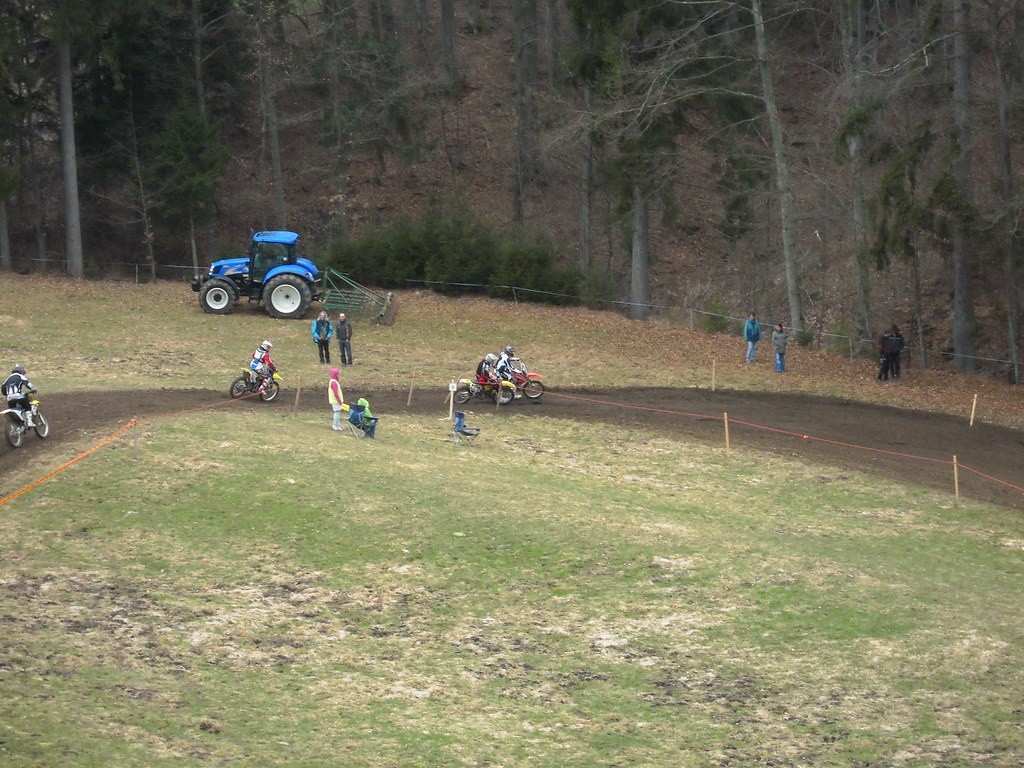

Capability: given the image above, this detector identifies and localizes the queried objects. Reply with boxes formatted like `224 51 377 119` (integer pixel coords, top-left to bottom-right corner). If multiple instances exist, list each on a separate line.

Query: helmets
260 340 270 353
504 344 516 358
11 364 24 375
486 353 499 367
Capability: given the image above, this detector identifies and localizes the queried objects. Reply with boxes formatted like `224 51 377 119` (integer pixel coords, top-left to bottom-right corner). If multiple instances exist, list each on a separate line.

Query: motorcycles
0 389 49 448
498 358 544 399
230 368 283 402
452 368 517 405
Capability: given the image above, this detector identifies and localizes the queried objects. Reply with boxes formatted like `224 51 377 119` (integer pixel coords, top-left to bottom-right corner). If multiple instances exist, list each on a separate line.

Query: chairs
451 411 481 448
348 402 379 439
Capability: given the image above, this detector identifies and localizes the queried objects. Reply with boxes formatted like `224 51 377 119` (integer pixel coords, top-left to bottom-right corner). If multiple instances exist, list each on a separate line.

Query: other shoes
332 425 342 431
341 363 346 366
349 363 352 367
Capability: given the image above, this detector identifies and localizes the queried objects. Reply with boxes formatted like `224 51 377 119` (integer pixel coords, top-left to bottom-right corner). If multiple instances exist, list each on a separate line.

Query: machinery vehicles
191 230 318 319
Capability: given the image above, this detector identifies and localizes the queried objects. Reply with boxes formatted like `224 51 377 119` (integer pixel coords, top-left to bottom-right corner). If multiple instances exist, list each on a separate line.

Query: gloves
31 387 37 394
273 367 278 372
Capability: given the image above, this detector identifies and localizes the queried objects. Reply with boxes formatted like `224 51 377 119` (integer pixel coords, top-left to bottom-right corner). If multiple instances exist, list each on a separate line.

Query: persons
743 313 762 363
328 368 345 431
493 345 523 400
1 364 36 427
358 394 375 439
772 323 788 372
476 353 508 403
250 340 278 395
876 325 905 383
336 312 353 367
311 311 333 365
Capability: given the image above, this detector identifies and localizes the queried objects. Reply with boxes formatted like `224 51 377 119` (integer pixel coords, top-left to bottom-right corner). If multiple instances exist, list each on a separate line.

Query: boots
25 411 36 428
258 379 268 395
